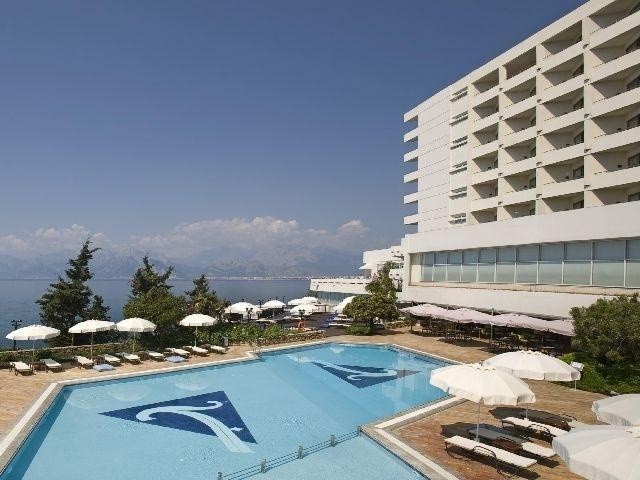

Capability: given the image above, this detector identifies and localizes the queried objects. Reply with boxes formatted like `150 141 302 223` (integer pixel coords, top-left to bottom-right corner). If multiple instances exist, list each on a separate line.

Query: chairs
519 408 589 428
445 435 538 477
502 416 569 443
468 427 557 460
10 342 226 374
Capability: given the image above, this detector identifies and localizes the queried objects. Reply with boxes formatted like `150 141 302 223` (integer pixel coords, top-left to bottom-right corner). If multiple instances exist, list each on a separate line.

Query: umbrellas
551 424 640 480
6 325 62 358
429 362 536 438
179 314 216 346
115 317 158 354
332 296 357 310
398 304 583 350
485 349 582 417
288 296 318 316
68 319 115 355
225 302 262 322
260 299 286 318
590 394 640 426
